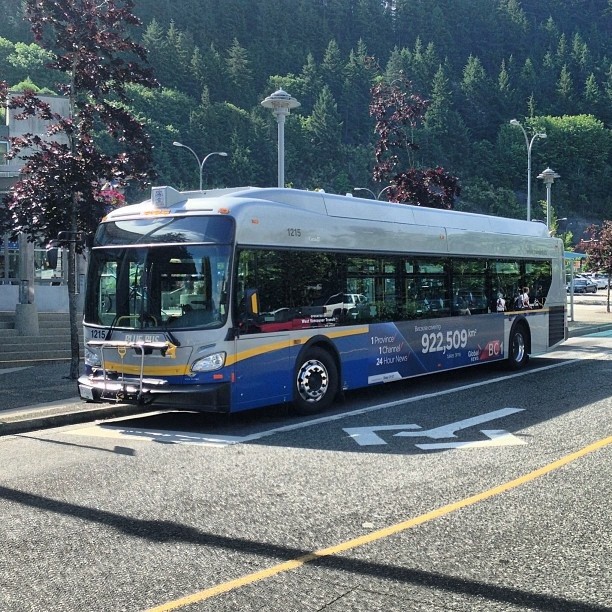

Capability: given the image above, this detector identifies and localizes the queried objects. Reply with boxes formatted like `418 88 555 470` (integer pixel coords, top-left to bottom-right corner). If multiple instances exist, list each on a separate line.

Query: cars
568 278 598 293
239 308 322 333
589 272 612 288
321 293 367 318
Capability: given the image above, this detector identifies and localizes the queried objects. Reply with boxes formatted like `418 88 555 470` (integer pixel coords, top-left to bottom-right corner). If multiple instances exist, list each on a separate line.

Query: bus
79 185 565 414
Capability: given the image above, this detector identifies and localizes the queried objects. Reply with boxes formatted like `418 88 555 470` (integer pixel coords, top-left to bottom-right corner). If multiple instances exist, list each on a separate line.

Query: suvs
566 272 596 289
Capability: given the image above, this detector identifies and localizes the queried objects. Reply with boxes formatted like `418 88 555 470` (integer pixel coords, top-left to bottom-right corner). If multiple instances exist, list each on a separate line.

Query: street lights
510 117 547 221
259 87 301 189
172 141 227 190
352 185 397 198
537 167 560 231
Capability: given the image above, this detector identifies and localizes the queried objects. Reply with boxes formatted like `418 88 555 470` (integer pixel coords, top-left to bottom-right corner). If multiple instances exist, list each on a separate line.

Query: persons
523 286 531 309
517 288 525 308
497 292 506 311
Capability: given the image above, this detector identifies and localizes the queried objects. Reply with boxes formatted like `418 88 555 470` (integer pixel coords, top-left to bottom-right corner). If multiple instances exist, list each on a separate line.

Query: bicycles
101 287 112 314
